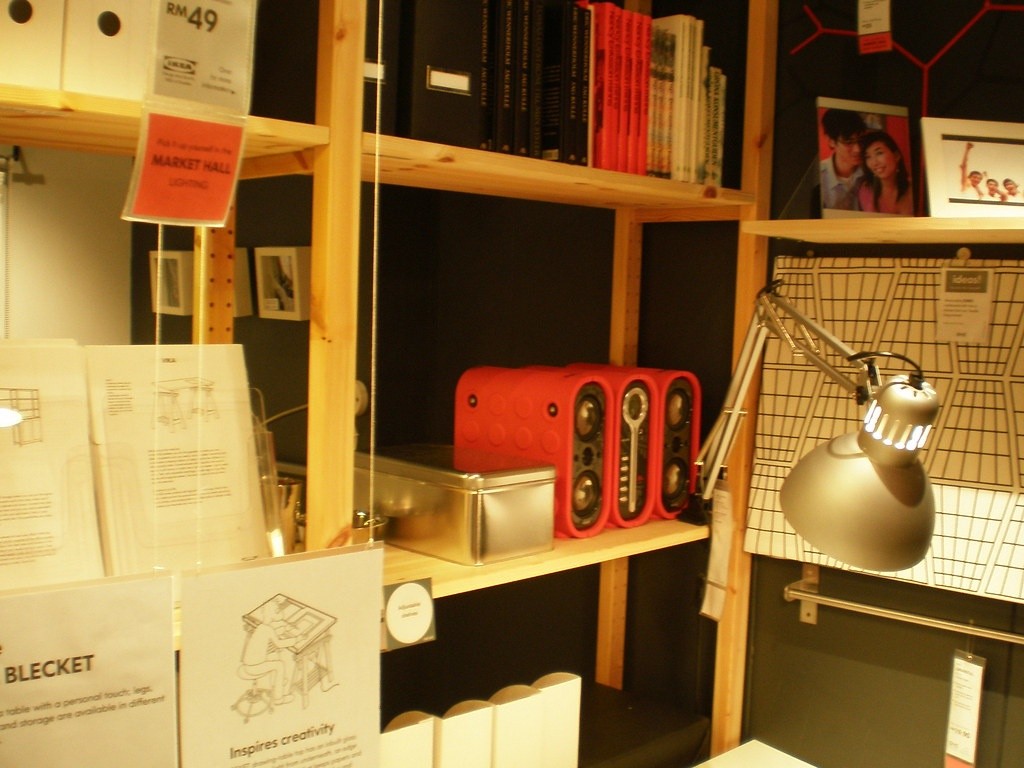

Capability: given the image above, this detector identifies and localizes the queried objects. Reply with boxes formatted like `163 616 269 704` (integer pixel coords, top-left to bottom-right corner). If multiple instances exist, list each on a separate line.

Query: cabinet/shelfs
0 0 1024 768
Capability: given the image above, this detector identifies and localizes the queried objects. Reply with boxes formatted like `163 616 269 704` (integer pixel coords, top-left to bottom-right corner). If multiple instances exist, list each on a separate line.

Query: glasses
838 139 861 146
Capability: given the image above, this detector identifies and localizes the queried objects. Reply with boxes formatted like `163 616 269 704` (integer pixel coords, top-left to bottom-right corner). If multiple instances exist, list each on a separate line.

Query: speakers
454 363 701 538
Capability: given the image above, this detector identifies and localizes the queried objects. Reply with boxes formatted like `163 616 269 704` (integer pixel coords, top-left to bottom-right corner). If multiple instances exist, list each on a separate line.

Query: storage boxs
353 446 557 567
0 1 257 114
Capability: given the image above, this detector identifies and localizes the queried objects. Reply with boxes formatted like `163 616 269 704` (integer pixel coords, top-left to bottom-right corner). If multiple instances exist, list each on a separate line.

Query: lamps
693 280 939 573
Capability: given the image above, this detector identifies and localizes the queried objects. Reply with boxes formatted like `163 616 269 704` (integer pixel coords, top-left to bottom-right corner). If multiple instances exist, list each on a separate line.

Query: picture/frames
920 118 1024 220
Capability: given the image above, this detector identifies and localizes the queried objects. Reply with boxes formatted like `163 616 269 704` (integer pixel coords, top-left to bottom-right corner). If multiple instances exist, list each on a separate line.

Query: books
252 2 728 189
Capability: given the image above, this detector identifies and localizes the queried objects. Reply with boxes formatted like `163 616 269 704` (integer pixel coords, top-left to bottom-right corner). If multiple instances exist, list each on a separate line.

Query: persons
818 108 910 216
954 143 1022 203
